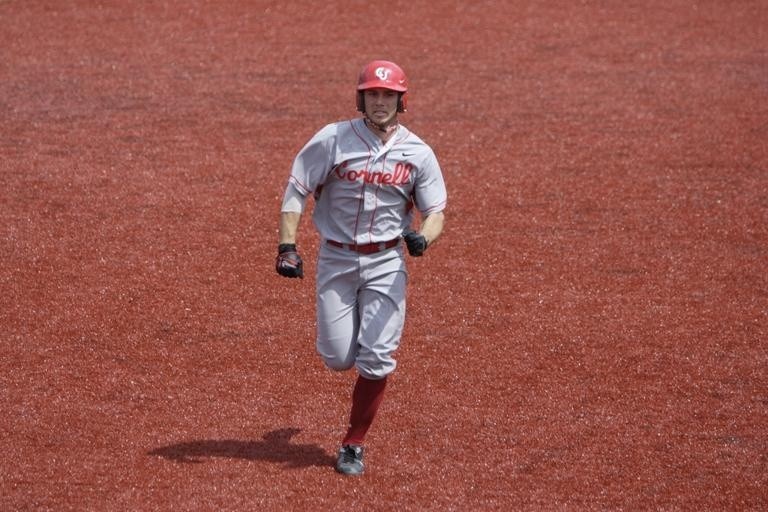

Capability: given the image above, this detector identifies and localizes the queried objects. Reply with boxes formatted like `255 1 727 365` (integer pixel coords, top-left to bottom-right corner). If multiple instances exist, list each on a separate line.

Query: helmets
355 60 407 112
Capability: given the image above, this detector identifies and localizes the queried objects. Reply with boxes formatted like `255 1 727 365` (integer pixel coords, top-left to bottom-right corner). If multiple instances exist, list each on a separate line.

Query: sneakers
337 444 364 475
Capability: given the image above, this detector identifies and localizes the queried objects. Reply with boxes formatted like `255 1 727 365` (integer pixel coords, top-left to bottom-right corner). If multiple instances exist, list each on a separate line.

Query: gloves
404 234 427 256
276 243 304 279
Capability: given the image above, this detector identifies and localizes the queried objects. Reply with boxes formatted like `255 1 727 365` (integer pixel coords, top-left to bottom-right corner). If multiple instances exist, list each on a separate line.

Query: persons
274 59 448 476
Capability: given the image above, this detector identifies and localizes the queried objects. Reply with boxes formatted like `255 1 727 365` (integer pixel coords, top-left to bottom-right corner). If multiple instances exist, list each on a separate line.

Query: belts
327 240 399 256
